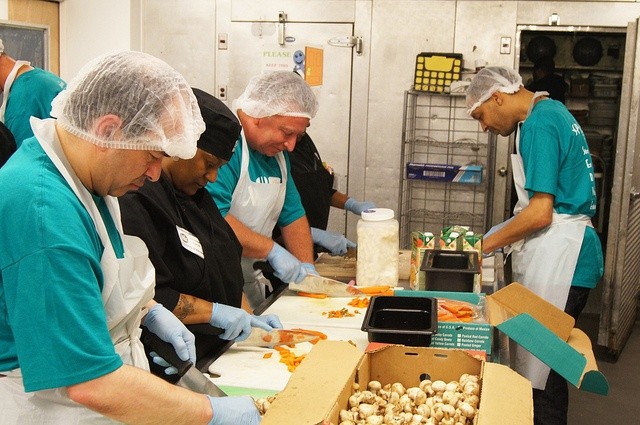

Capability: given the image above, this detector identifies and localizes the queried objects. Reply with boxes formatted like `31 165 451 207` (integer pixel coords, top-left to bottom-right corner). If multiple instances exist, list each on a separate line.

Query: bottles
354 208 402 288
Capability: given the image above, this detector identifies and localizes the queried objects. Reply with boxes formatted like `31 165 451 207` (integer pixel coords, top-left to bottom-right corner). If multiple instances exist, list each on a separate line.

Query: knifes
184 324 319 347
252 261 362 296
315 245 358 258
143 333 228 397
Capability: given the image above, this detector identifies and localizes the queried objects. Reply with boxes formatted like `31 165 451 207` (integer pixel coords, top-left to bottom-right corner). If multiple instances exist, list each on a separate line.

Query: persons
114 86 285 385
0 52 261 424
203 69 319 315
266 71 377 299
1 122 18 169
0 40 67 149
465 64 605 425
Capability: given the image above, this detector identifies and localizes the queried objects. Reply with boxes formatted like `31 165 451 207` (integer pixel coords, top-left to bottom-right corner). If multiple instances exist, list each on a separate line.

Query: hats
192 88 242 161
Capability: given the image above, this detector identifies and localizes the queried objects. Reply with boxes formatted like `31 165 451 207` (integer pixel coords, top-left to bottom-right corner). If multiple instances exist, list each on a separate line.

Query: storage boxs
257 341 536 425
394 283 608 395
360 295 437 381
420 249 480 293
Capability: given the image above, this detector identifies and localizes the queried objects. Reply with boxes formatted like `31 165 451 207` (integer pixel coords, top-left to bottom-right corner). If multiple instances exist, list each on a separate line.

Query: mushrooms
337 372 481 425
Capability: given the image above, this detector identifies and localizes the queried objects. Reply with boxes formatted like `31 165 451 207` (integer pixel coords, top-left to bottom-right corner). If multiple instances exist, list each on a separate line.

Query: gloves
210 302 272 343
300 263 320 276
208 395 260 425
344 199 374 215
311 228 356 256
267 241 306 284
250 314 283 329
143 304 196 375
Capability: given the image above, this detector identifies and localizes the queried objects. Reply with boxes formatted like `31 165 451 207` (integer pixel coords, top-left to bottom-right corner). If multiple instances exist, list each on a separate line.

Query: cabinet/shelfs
396 84 497 252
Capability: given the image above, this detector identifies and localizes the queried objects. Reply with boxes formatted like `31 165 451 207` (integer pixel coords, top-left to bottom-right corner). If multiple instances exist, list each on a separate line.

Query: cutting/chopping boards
482 252 496 287
314 249 412 281
203 323 369 400
260 295 372 329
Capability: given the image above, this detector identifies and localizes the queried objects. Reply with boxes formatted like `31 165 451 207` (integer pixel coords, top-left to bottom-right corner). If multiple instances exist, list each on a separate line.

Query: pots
572 38 603 66
526 36 557 63
